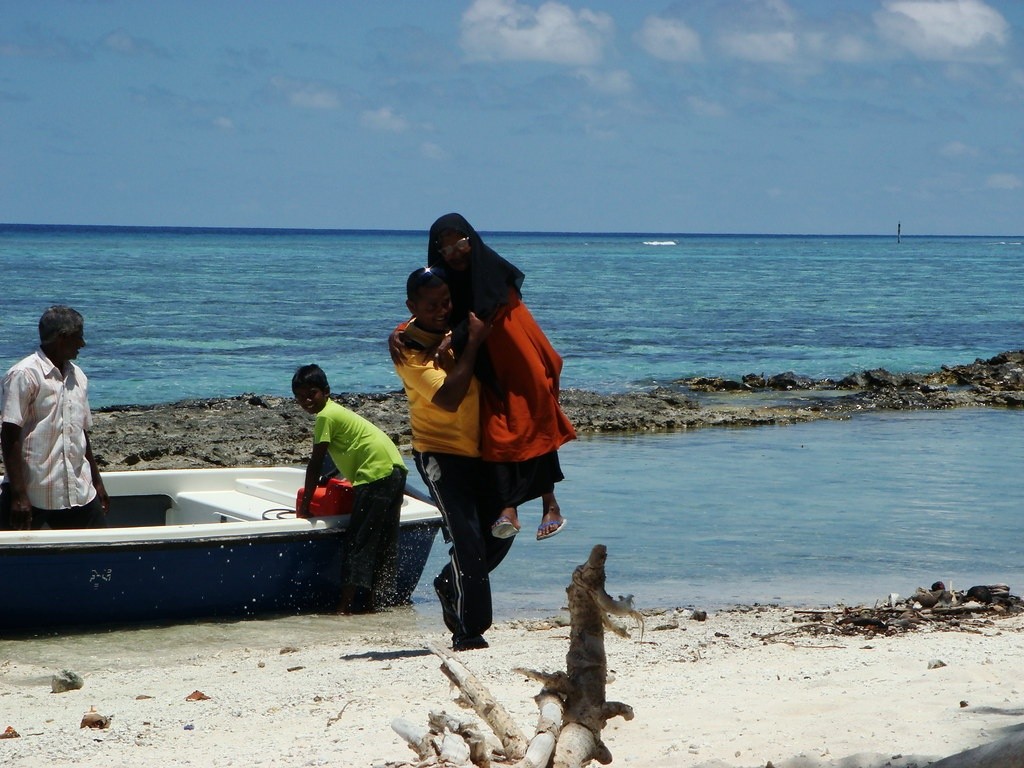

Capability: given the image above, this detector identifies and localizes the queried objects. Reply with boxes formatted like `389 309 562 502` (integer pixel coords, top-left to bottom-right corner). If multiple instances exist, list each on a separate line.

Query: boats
0 465 442 628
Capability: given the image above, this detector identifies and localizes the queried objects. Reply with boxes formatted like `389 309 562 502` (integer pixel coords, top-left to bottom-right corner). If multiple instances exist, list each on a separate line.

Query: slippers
535 516 568 540
491 515 520 540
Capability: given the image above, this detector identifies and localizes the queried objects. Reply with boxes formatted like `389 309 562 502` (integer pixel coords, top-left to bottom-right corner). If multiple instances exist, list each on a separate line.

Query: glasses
437 236 470 257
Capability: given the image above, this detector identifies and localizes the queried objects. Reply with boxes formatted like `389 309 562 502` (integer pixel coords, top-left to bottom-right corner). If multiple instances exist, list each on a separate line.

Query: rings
434 353 439 358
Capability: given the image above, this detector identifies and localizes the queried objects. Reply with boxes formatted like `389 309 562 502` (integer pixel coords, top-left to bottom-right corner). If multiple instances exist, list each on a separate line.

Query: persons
388 213 575 540
392 267 519 652
0 306 110 531
291 364 408 615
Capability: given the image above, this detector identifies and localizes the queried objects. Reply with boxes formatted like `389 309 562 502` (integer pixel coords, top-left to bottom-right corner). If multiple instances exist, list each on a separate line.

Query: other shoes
433 576 461 635
451 633 489 651
